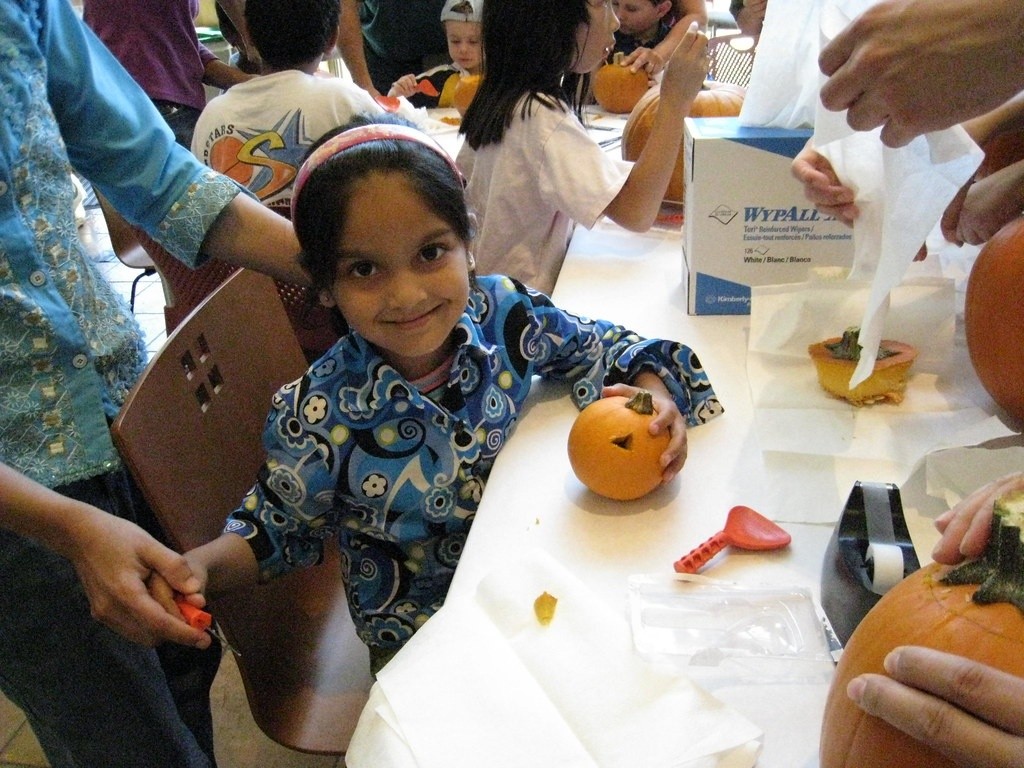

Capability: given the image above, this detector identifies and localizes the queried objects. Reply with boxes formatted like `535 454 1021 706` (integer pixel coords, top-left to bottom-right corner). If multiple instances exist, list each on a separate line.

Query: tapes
866 544 904 591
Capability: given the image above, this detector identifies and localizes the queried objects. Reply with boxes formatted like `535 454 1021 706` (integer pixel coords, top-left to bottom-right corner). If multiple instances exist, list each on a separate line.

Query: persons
844 467 1024 768
1 0 321 767
791 0 1024 261
148 117 726 683
82 0 712 297
0 0 314 768
730 0 770 35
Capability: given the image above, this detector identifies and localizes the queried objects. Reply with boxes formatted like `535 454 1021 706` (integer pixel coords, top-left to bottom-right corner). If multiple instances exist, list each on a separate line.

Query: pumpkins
818 477 1023 767
592 51 650 113
620 73 746 202
965 211 1024 425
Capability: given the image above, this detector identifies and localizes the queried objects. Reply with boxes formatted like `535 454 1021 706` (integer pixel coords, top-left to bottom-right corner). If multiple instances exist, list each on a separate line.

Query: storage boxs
685 117 893 316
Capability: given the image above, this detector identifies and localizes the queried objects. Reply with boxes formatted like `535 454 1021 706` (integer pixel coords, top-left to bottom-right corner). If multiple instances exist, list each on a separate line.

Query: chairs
96 186 377 755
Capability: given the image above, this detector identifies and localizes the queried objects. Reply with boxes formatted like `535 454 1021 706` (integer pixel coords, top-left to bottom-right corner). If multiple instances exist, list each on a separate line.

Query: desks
343 108 1024 768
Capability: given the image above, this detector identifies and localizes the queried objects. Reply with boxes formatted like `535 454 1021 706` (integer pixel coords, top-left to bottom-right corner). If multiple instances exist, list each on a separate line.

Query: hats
440 0 484 22
